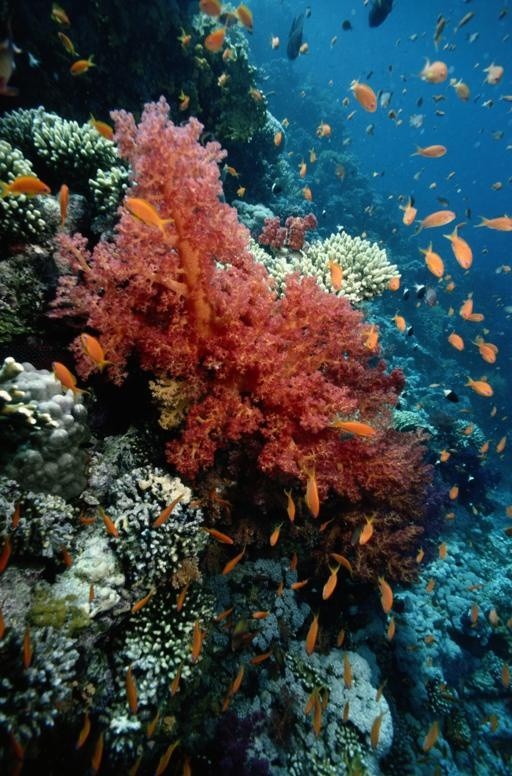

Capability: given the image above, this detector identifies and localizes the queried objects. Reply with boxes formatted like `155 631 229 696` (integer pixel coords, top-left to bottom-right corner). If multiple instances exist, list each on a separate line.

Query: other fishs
0 0 510 776
